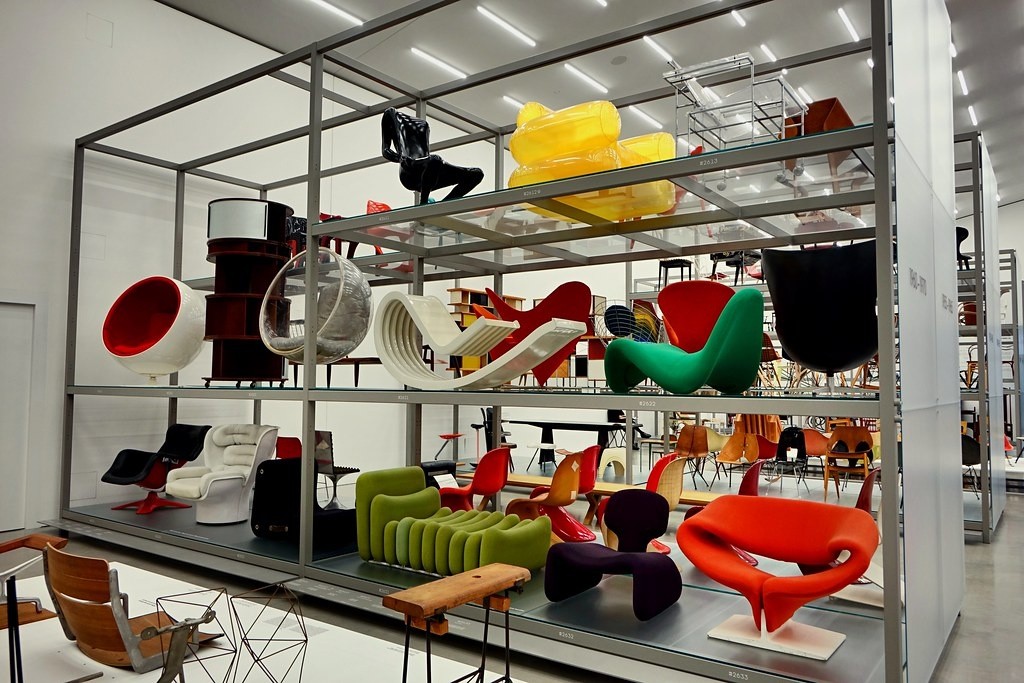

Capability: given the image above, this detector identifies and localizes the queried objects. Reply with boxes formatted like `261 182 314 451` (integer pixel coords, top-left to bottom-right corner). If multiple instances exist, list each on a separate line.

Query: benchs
456 474 736 526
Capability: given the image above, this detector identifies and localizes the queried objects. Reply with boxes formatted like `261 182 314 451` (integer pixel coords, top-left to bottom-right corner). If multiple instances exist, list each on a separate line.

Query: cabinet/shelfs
201 197 294 389
38 0 1024 683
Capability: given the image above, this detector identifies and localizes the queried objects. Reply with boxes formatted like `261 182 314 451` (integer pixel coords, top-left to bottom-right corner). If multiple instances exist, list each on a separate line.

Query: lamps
312 0 1002 237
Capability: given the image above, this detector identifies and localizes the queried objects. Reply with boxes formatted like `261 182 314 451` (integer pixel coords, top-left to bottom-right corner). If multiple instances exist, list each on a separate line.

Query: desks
638 440 678 472
508 421 643 468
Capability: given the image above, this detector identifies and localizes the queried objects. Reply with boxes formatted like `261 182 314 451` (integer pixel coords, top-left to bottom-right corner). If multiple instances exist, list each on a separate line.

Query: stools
526 443 558 473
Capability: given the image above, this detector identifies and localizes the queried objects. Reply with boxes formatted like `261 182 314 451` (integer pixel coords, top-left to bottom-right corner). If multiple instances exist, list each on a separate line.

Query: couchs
356 466 552 576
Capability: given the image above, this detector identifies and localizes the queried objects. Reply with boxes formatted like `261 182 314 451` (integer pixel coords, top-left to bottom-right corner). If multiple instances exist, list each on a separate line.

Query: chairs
0 54 1014 683
